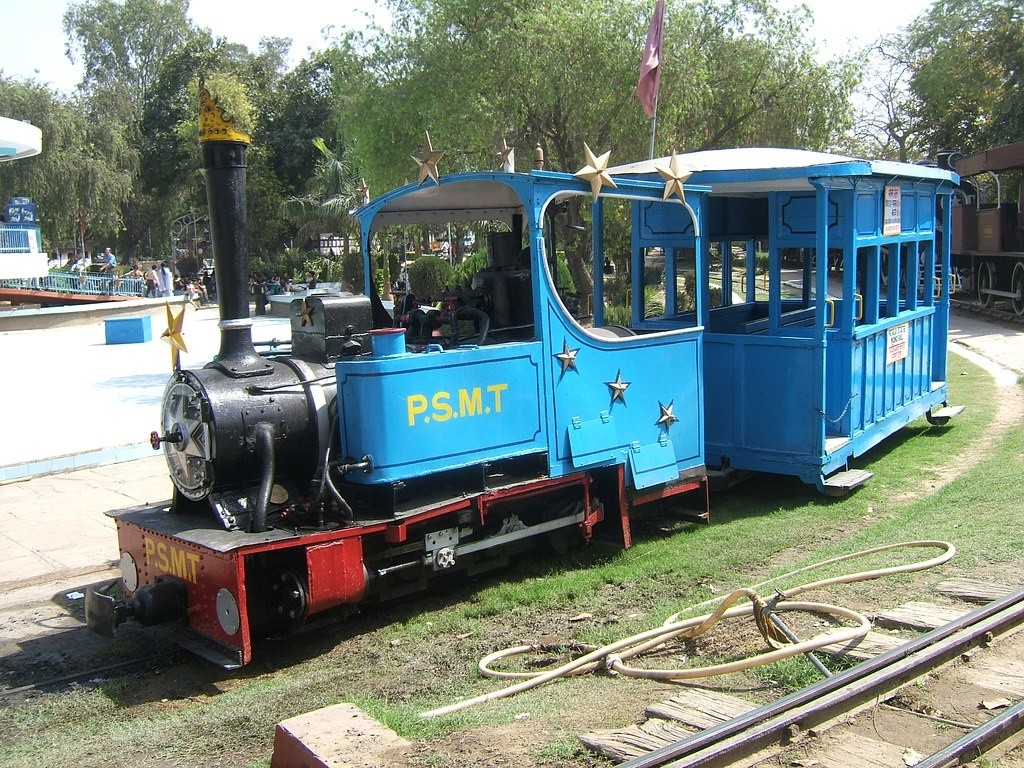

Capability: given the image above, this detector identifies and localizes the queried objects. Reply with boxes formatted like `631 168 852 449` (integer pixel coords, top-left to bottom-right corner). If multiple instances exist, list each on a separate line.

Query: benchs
738 308 816 335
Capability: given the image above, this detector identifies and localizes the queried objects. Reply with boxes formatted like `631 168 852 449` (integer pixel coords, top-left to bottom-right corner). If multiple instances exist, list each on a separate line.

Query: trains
801 139 1023 319
98 82 964 677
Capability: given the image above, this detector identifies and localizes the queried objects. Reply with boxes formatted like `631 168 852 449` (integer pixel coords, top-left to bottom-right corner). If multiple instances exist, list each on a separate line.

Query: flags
635 2 665 118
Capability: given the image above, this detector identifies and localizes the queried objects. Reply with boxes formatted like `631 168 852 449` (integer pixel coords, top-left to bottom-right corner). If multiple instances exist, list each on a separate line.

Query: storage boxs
104 314 152 344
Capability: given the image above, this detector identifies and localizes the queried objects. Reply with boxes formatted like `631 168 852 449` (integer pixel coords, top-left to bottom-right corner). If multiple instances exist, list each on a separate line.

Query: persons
305 270 317 289
121 262 217 311
70 254 83 290
249 270 289 316
99 248 117 295
